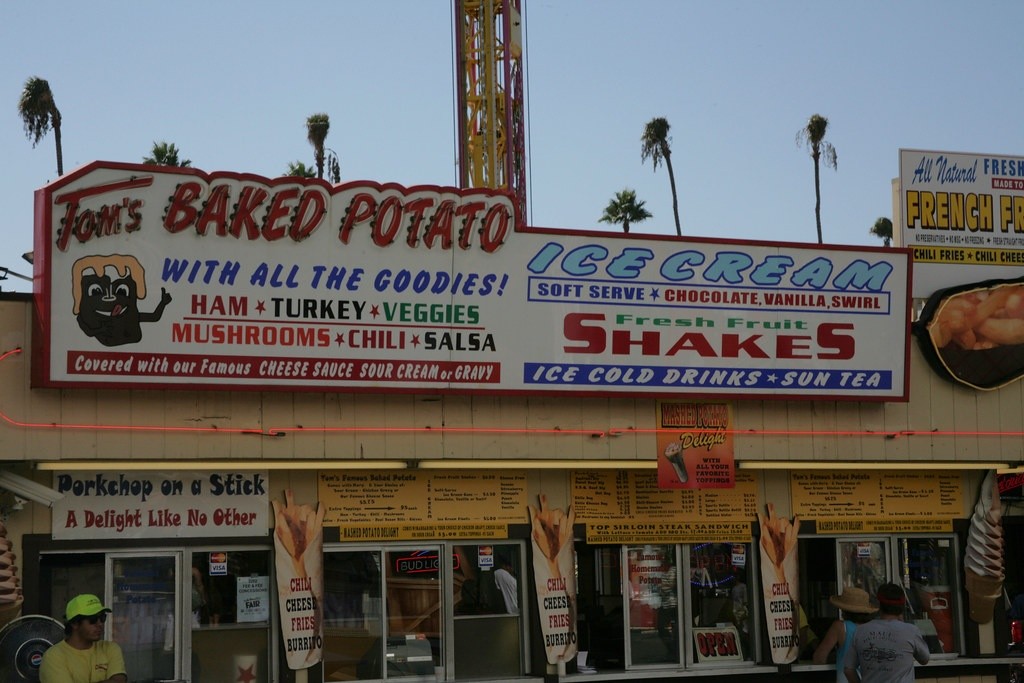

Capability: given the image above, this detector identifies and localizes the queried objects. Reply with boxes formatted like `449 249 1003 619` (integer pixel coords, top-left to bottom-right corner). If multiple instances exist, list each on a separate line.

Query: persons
844 584 929 683
454 579 502 615
39 593 128 683
799 586 879 683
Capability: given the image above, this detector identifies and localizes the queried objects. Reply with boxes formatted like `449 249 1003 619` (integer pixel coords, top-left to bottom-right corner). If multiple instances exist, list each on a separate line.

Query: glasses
81 613 107 625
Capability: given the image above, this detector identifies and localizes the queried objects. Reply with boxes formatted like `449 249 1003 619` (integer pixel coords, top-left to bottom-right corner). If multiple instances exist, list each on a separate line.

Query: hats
834 587 880 613
66 593 113 621
